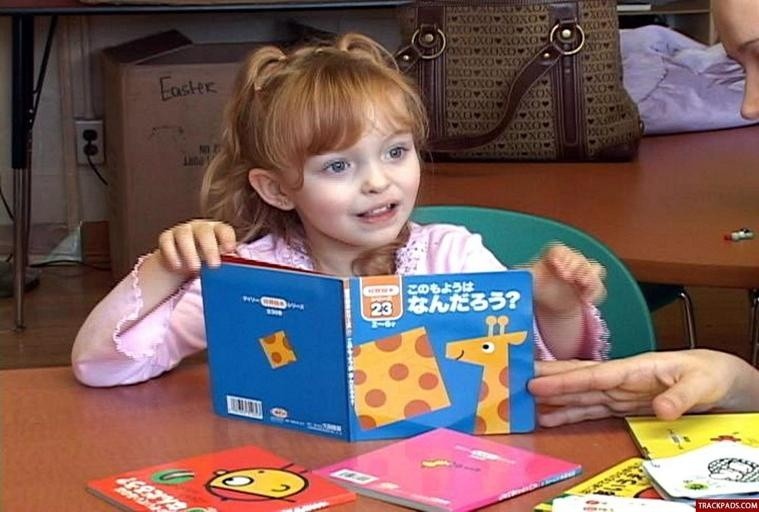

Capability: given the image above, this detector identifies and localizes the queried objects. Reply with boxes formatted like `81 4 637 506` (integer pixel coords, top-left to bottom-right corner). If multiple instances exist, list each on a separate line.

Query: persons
68 33 619 389
527 0 758 428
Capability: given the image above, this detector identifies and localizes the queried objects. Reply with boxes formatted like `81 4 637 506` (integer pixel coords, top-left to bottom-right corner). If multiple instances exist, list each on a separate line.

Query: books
187 250 537 441
85 414 757 511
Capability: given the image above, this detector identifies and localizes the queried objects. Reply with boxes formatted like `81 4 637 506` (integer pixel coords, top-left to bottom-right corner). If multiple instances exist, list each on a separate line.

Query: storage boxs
99 19 339 284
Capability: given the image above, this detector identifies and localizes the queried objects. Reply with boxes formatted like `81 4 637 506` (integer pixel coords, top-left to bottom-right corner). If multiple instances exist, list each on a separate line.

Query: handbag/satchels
388 2 642 160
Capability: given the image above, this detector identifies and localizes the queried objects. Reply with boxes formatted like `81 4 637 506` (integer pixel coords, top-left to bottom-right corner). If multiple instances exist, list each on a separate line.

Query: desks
1 1 712 331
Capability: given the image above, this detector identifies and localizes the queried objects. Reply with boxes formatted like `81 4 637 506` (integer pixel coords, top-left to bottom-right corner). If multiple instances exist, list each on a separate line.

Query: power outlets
74 115 106 170
